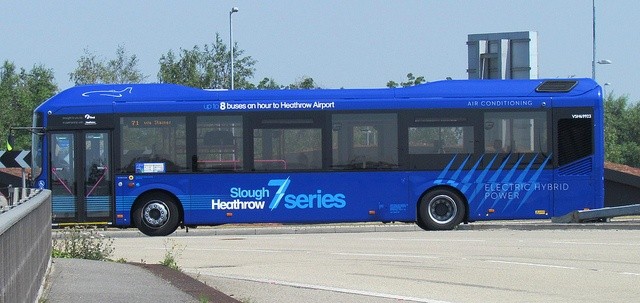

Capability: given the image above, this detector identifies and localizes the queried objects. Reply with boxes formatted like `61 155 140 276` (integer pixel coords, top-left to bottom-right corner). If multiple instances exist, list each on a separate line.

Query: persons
493 140 505 154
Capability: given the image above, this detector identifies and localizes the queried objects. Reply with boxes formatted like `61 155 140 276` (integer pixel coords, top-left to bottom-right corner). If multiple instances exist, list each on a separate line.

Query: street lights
229 7 238 170
592 58 611 81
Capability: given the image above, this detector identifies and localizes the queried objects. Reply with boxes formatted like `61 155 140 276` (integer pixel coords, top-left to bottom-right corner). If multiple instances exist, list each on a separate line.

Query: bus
6 77 606 236
